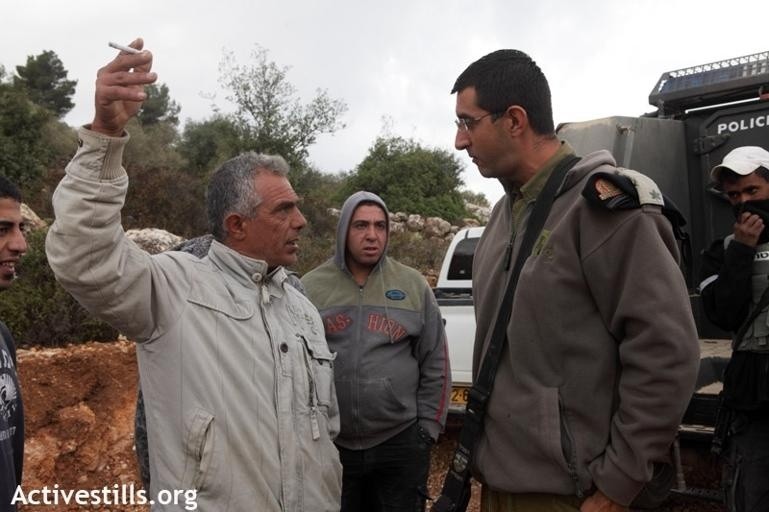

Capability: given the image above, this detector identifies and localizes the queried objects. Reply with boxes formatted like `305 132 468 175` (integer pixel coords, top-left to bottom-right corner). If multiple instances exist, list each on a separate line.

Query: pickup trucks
430 226 486 413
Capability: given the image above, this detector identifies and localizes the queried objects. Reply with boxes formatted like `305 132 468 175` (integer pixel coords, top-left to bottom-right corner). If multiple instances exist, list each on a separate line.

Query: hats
710 146 769 183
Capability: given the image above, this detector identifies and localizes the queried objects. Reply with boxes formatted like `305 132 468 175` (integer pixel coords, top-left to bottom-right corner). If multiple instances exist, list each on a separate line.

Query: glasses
454 113 490 132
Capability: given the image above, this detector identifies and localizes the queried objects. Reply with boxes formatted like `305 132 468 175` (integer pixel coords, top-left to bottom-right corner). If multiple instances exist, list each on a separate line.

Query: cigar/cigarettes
105 41 142 56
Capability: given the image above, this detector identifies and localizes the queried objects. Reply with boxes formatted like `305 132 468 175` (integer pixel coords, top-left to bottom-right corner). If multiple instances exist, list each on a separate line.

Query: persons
302 190 451 511
130 232 307 509
42 35 343 511
695 142 768 508
0 170 29 511
448 46 702 512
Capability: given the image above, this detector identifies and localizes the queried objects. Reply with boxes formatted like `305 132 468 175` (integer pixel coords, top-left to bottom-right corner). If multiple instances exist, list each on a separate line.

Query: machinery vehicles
555 49 769 500
